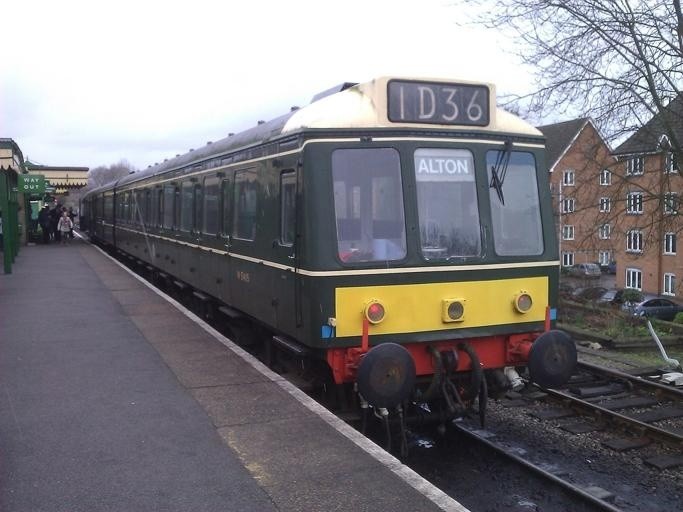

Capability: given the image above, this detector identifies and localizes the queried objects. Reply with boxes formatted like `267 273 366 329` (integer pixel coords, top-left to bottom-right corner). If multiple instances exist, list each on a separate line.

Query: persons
37 201 79 245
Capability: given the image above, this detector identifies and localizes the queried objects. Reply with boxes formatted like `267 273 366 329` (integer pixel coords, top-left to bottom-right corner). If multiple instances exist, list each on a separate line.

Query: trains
79 77 577 427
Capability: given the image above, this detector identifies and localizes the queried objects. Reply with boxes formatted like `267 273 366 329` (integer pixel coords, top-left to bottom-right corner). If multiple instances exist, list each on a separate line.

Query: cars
607 261 616 274
570 287 683 317
567 262 602 280
